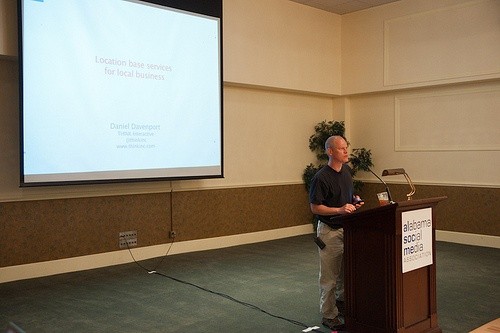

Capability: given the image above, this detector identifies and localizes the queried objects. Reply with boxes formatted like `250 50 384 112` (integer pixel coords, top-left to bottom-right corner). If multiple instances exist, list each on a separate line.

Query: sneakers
336 301 344 318
321 317 344 331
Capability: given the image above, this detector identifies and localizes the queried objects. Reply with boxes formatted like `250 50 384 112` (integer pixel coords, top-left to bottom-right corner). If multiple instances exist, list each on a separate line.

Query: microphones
354 158 396 205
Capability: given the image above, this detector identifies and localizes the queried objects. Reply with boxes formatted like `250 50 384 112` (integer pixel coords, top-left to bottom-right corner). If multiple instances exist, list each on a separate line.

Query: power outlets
119 231 137 248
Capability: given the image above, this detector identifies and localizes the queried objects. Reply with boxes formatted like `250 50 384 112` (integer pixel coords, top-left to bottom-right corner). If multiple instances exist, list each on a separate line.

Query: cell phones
354 199 365 206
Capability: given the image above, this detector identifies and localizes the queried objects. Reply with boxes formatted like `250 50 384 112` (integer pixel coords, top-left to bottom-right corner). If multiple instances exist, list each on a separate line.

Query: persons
308 136 364 331
